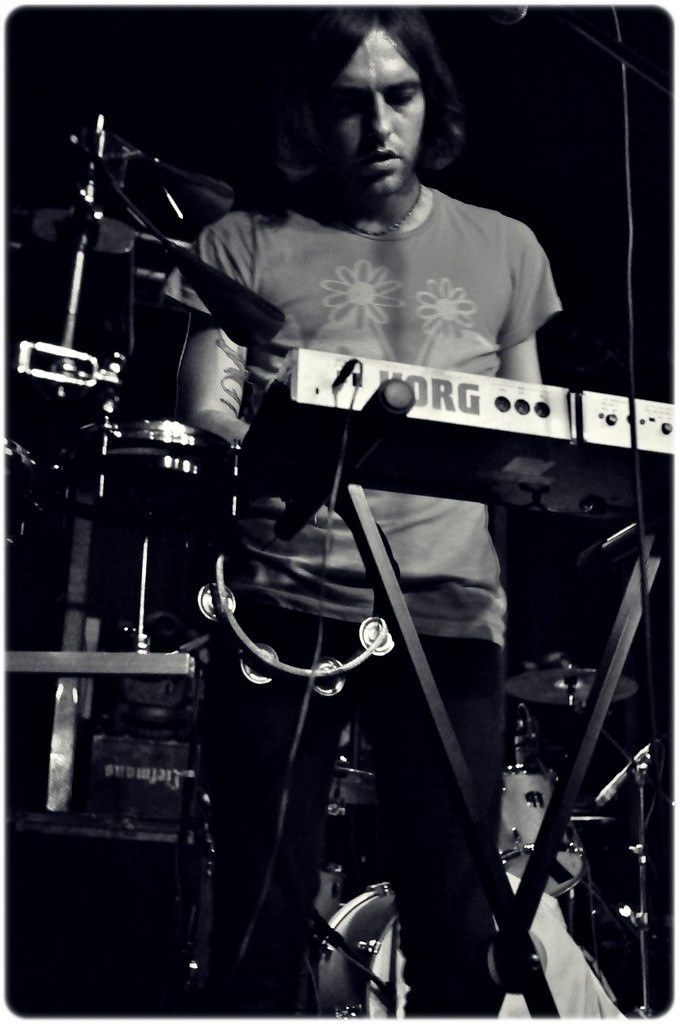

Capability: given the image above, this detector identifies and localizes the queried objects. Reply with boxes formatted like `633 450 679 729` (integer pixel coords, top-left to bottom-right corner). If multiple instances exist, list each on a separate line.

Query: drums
492 771 586 897
312 881 413 1017
308 859 346 924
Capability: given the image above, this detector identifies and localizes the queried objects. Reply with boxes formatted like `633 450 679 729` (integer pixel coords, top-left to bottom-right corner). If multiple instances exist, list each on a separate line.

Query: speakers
5 810 213 1020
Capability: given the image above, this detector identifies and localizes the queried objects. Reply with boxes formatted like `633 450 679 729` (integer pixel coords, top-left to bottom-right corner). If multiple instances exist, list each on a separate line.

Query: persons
156 7 564 1017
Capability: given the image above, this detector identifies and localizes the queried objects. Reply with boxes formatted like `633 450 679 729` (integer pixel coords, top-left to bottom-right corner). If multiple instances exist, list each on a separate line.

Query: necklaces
318 181 422 238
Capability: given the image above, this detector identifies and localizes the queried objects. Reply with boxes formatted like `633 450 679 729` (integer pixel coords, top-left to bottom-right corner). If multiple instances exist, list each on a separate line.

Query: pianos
219 340 673 530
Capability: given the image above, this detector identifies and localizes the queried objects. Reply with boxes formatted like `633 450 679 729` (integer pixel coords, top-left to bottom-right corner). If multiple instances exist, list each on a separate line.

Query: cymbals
501 663 639 707
205 551 397 699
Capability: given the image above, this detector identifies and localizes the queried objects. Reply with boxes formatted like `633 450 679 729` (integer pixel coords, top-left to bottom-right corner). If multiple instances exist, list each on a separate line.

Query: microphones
488 6 528 25
514 708 526 766
594 743 652 807
